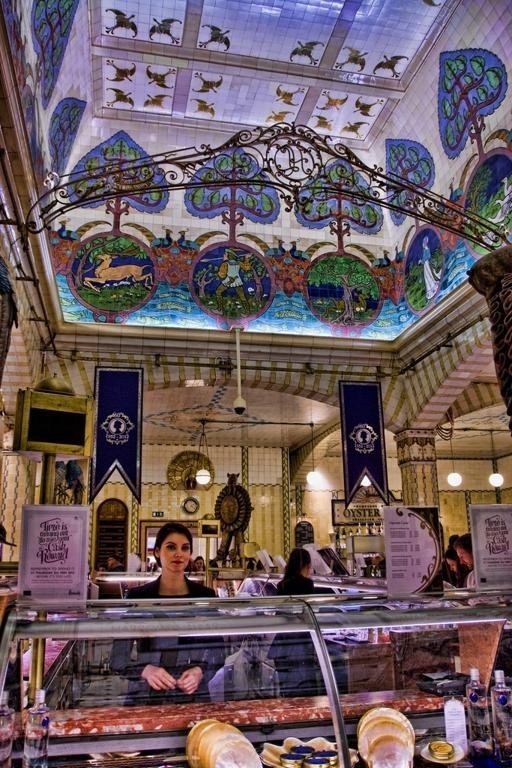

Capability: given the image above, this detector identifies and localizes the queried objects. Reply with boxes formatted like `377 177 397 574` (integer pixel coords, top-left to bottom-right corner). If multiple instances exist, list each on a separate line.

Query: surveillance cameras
233 397 247 415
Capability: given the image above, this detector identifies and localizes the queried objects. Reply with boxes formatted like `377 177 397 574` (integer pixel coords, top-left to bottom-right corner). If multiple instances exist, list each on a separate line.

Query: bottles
0 691 14 767
23 688 49 768
465 665 490 744
491 670 511 756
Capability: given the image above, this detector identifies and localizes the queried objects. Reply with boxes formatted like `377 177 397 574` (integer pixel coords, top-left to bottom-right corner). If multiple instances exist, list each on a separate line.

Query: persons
192 555 273 573
137 558 161 572
99 553 125 599
366 551 386 578
423 531 475 591
265 545 321 697
108 522 225 708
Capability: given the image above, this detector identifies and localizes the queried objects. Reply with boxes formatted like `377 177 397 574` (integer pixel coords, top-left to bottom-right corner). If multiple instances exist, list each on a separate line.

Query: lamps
232 325 247 415
193 420 212 487
444 431 504 489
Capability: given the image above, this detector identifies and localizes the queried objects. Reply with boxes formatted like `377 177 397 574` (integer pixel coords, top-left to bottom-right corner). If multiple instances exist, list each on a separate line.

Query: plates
356 706 415 767
184 719 262 768
420 741 465 764
